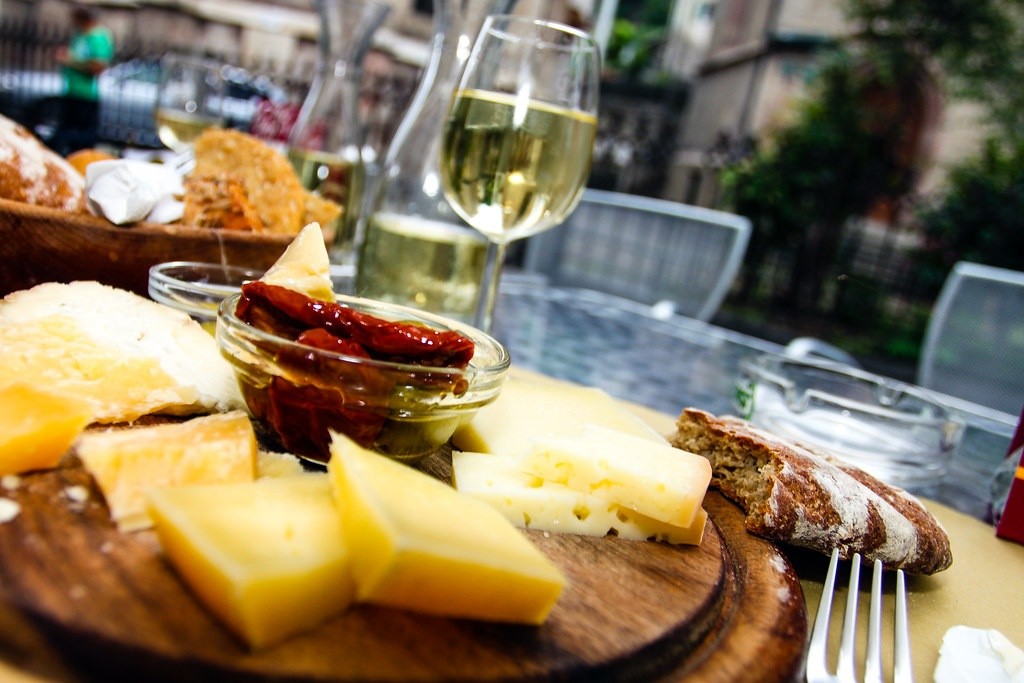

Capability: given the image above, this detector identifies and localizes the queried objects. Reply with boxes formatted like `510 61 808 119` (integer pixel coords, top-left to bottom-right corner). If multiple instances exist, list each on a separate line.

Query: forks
804 547 914 682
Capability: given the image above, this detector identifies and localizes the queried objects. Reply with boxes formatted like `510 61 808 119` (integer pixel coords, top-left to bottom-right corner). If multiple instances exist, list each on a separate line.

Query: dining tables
321 257 1024 545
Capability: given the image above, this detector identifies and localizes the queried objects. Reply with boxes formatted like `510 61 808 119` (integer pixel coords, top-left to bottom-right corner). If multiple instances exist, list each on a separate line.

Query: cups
157 52 224 152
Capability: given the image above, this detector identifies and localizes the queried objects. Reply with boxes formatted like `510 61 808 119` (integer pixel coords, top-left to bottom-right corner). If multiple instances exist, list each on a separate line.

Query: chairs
911 260 1024 415
508 187 752 325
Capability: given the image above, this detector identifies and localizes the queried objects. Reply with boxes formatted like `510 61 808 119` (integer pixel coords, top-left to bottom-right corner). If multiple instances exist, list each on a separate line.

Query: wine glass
441 11 601 339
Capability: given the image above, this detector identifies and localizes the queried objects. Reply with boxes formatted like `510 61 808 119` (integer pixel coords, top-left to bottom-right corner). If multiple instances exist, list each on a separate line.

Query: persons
45 11 115 156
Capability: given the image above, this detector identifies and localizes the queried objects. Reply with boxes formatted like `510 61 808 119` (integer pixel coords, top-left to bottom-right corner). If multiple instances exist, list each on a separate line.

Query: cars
0 49 329 160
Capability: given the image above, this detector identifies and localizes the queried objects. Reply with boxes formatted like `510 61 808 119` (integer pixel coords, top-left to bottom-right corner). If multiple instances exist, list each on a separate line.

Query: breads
671 407 952 574
0 113 342 235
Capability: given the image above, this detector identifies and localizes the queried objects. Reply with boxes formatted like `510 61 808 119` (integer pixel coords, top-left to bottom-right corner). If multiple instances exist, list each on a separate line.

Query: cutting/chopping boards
0 385 802 682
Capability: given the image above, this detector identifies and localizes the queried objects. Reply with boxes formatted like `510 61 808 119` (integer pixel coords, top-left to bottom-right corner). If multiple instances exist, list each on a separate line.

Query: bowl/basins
0 196 334 297
147 260 263 322
215 290 511 473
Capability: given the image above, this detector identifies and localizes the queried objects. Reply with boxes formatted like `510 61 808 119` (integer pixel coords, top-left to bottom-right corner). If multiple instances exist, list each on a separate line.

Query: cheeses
441 382 712 549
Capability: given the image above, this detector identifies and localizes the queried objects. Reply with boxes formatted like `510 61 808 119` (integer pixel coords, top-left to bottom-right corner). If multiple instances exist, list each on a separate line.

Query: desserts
1 274 561 640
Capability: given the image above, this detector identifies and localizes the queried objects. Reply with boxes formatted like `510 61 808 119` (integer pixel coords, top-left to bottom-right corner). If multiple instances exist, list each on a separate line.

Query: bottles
356 0 510 319
288 0 390 243
